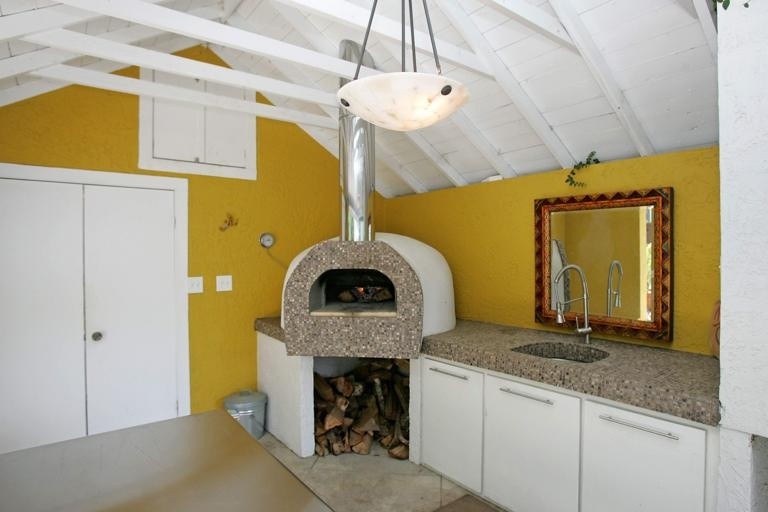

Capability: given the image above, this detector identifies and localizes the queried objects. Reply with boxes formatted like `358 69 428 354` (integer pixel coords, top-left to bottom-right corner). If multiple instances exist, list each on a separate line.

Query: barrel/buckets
222 388 269 441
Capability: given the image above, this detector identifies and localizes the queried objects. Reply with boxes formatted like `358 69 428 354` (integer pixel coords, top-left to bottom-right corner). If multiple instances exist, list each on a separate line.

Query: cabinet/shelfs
137 66 257 180
584 381 707 511
419 354 485 500
483 365 582 510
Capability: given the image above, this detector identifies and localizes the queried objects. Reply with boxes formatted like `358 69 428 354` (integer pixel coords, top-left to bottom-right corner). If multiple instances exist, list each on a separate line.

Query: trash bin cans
224 391 266 440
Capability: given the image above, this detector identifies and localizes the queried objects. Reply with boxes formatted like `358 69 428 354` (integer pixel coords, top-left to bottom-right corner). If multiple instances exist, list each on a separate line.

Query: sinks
509 339 612 365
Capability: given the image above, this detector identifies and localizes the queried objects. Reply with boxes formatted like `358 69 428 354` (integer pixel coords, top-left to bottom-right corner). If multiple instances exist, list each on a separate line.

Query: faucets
606 258 624 318
553 263 592 345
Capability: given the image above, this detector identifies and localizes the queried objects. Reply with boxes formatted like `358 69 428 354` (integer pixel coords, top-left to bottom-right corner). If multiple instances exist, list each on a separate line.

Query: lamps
337 0 470 133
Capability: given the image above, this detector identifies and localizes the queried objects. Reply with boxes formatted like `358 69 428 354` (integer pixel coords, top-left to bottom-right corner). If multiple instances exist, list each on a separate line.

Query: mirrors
532 186 674 342
